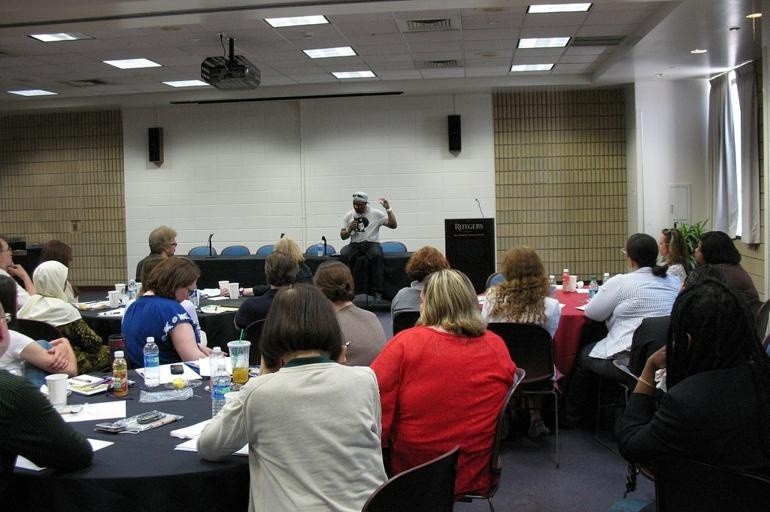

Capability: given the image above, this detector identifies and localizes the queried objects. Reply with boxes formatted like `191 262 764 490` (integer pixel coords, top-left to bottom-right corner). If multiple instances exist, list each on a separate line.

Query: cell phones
169 364 184 374
135 410 167 424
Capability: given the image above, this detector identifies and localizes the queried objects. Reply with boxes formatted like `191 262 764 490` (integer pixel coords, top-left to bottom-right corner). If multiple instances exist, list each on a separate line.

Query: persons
340 191 397 302
0 227 513 511
569 228 769 511
476 247 561 450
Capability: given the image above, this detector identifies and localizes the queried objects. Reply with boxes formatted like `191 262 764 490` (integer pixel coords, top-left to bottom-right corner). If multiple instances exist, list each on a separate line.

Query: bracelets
386 207 392 213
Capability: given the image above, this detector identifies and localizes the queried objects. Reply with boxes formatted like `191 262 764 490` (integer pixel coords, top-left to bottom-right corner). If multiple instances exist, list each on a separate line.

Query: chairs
221 246 250 255
256 245 274 256
17 319 62 342
755 298 770 342
393 311 421 336
306 245 335 256
485 322 560 469
486 273 505 287
381 242 407 253
188 246 217 255
362 445 460 512
595 316 669 432
244 319 266 361
463 367 525 512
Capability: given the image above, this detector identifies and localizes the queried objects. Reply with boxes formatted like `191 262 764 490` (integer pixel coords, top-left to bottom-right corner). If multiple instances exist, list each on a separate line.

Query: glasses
353 194 367 201
1 247 12 252
621 248 628 255
171 243 177 246
0 313 12 322
105 383 139 400
184 286 194 297
660 243 669 245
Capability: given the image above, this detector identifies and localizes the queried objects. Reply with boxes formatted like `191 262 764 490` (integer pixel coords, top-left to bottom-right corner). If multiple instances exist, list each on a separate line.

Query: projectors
200 54 261 90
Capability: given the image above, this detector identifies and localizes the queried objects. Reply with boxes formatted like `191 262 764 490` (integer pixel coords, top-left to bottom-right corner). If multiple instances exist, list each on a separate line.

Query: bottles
602 272 610 283
143 337 161 388
128 278 138 300
112 350 129 397
211 364 233 418
209 346 228 400
548 275 557 298
562 269 570 293
589 275 599 301
317 242 324 257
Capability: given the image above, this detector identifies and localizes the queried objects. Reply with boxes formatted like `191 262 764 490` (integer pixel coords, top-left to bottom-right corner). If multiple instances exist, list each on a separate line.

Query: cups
229 283 239 300
107 291 120 308
570 276 577 293
227 340 251 384
44 373 69 407
218 281 229 292
114 283 126 297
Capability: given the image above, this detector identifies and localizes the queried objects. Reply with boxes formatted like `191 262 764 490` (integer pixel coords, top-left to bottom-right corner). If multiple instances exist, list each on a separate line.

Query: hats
353 192 369 203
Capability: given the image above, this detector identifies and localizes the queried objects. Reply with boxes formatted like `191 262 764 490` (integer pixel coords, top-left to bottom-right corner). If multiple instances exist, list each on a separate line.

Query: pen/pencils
72 378 92 382
345 341 351 347
57 412 78 414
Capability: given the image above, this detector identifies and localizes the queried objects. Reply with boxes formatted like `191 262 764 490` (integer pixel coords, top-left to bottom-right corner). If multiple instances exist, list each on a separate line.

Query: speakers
148 127 162 163
447 114 462 152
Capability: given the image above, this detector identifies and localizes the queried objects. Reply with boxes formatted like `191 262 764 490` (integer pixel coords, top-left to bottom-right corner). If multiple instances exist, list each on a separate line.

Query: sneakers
374 293 382 300
528 420 551 436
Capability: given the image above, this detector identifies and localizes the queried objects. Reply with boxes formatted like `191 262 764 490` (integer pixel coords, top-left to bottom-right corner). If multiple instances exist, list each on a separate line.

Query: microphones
321 235 328 254
206 233 215 257
278 231 285 238
474 198 485 217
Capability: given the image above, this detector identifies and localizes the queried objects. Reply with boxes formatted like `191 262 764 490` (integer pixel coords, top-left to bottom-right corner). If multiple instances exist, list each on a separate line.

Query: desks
184 253 411 308
80 289 253 356
12 369 249 511
476 286 592 371
